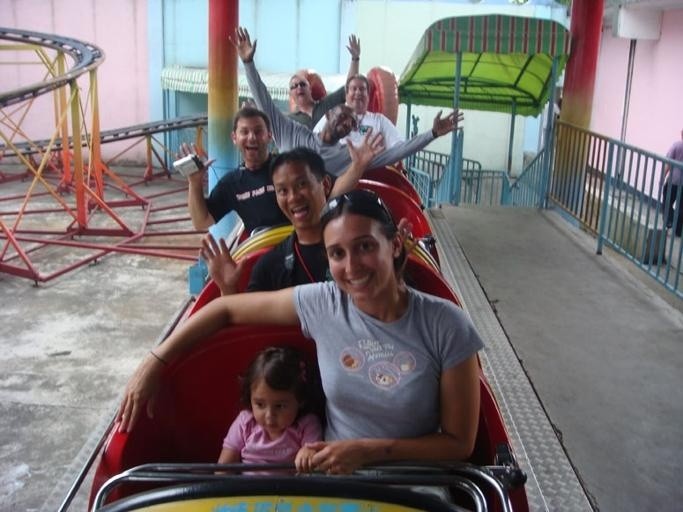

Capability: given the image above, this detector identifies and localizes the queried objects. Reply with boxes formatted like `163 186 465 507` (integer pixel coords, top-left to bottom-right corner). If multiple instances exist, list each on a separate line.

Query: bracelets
149 349 170 365
351 57 360 62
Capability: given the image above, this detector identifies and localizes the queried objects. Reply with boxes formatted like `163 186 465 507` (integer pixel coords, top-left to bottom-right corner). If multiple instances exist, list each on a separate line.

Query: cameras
173 155 204 178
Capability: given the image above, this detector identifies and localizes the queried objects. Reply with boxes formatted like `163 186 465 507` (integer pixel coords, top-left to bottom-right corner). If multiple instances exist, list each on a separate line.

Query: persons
196 145 421 298
114 189 486 475
214 344 324 475
553 87 562 118
543 98 561 147
173 108 386 237
658 129 682 238
227 26 466 177
240 33 361 132
312 74 405 150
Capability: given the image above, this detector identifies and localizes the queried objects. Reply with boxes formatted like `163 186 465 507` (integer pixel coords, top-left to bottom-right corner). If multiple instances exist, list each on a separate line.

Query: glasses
318 188 398 231
290 81 305 89
